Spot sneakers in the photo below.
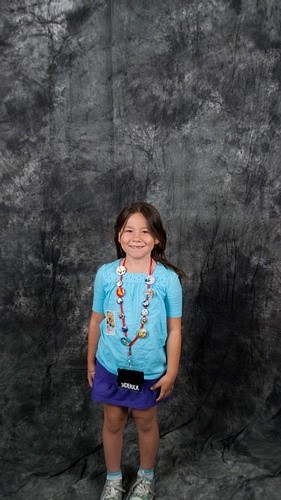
[100,475,125,500]
[127,478,155,500]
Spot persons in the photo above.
[88,201,192,500]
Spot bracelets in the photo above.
[87,371,96,373]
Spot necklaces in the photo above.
[115,256,155,355]
